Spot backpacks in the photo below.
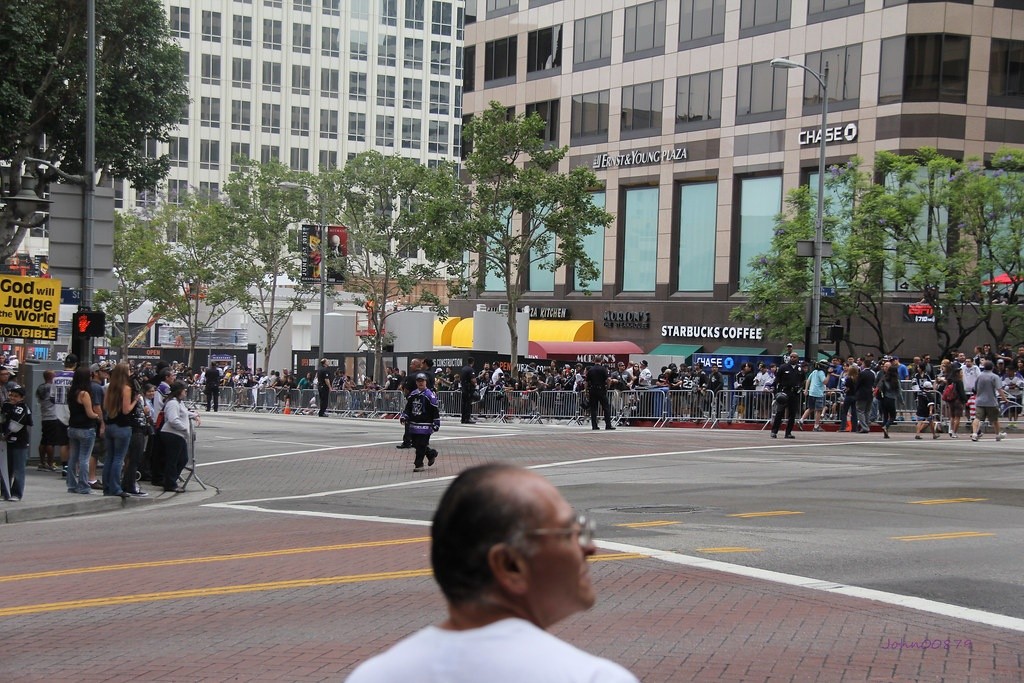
[942,380,957,402]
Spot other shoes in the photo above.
[396,443,411,449]
[948,430,958,438]
[414,466,425,472]
[971,433,979,441]
[460,419,476,424]
[969,432,983,437]
[915,435,923,439]
[428,452,438,466]
[317,411,328,417]
[60,471,186,498]
[0,495,21,502]
[769,422,889,438]
[933,435,940,439]
[592,426,616,430]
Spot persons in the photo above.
[987,286,1019,304]
[348,461,642,683]
[0,342,1024,502]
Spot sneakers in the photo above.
[996,432,1008,442]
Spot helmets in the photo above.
[574,363,583,369]
[776,393,789,406]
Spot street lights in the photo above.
[277,180,325,364]
[770,56,830,365]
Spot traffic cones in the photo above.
[283,399,290,415]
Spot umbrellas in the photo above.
[983,273,1024,291]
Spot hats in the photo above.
[866,353,899,361]
[435,368,442,374]
[65,353,111,373]
[640,360,648,366]
[787,342,793,347]
[416,373,428,381]
[923,381,933,389]
[7,381,25,396]
[997,359,1005,364]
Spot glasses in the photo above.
[621,365,625,366]
[528,516,595,549]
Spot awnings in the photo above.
[433,317,594,348]
[781,349,835,361]
[712,347,768,355]
[647,344,703,366]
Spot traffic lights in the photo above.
[827,324,844,341]
[72,310,105,338]
